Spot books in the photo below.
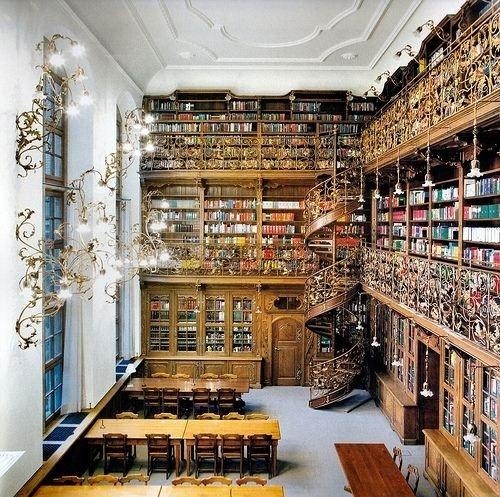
[149,197,315,270]
[147,100,375,135]
[149,299,251,351]
[444,346,500,484]
[377,173,500,268]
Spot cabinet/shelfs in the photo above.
[360,0,500,497]
[137,89,379,388]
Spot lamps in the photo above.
[374,70,397,86]
[420,338,435,398]
[393,146,405,195]
[422,126,436,188]
[358,165,365,203]
[391,318,402,367]
[373,156,383,199]
[289,91,296,101]
[467,102,484,177]
[463,368,483,448]
[412,19,452,44]
[393,44,420,64]
[345,91,354,101]
[14,107,183,350]
[224,93,232,101]
[363,85,388,102]
[14,33,96,178]
[370,305,381,347]
[356,293,364,329]
[169,94,177,101]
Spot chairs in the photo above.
[31,372,284,497]
[393,446,403,472]
[406,464,421,495]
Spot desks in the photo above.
[334,443,416,497]
